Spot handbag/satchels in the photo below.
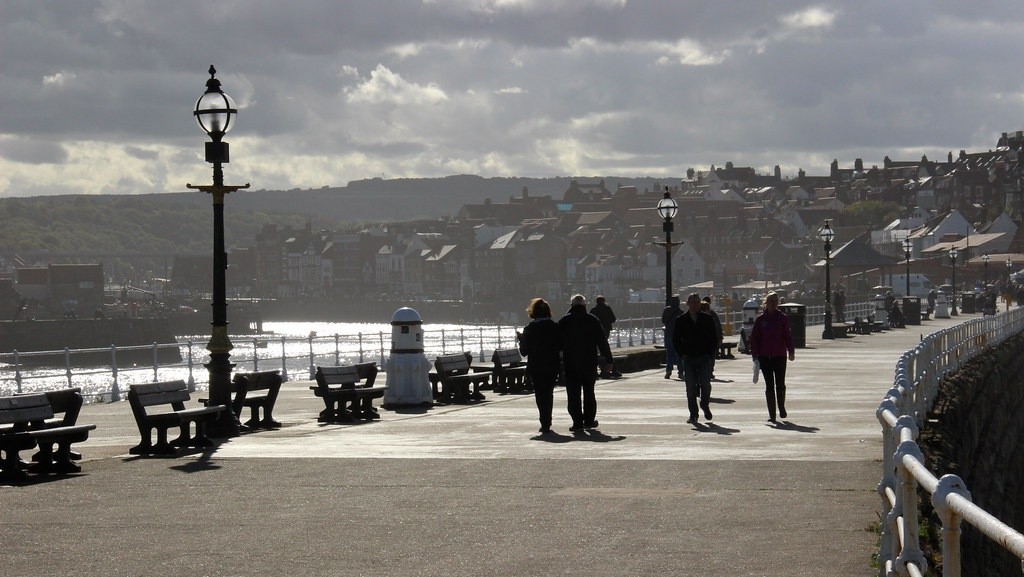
[753,359,760,384]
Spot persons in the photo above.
[662,293,722,423]
[589,297,616,339]
[750,292,795,422]
[884,279,1024,328]
[832,290,847,323]
[517,297,558,432]
[556,294,613,431]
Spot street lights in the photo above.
[652,185,683,308]
[902,236,914,297]
[186,62,251,438]
[949,245,958,316]
[819,219,836,340]
[1006,256,1013,306]
[983,250,990,292]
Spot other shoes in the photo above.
[687,414,698,423]
[539,422,550,433]
[612,370,622,377]
[678,371,684,379]
[711,373,715,379]
[569,425,583,431]
[584,419,598,429]
[665,371,671,378]
[700,401,712,420]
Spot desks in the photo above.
[470,348,510,391]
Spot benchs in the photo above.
[128,380,227,458]
[314,366,390,424]
[428,350,472,400]
[844,314,884,335]
[716,342,738,359]
[0,392,97,486]
[0,388,83,470]
[921,307,933,320]
[494,348,535,394]
[310,361,378,417]
[196,370,283,432]
[433,354,493,404]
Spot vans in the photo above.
[879,274,938,298]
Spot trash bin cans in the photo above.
[902,296,921,325]
[778,304,806,348]
[962,293,975,314]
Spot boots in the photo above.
[765,390,776,423]
[776,386,787,418]
